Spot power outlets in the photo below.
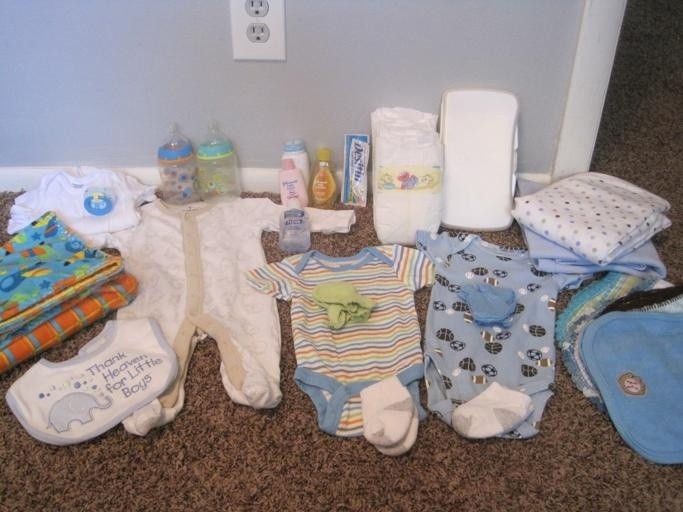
[229,0,286,60]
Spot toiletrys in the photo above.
[309,149,338,210]
[280,159,308,208]
[279,198,310,252]
